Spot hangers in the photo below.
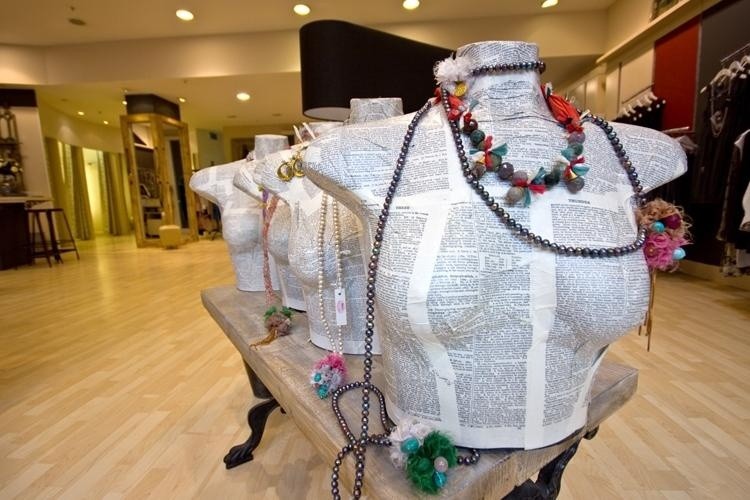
[659,127,699,155]
[612,83,667,121]
[700,43,750,94]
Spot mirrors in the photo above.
[119,113,199,248]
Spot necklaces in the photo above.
[331,61,647,499]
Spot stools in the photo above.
[25,208,79,268]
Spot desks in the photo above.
[201,286,639,500]
